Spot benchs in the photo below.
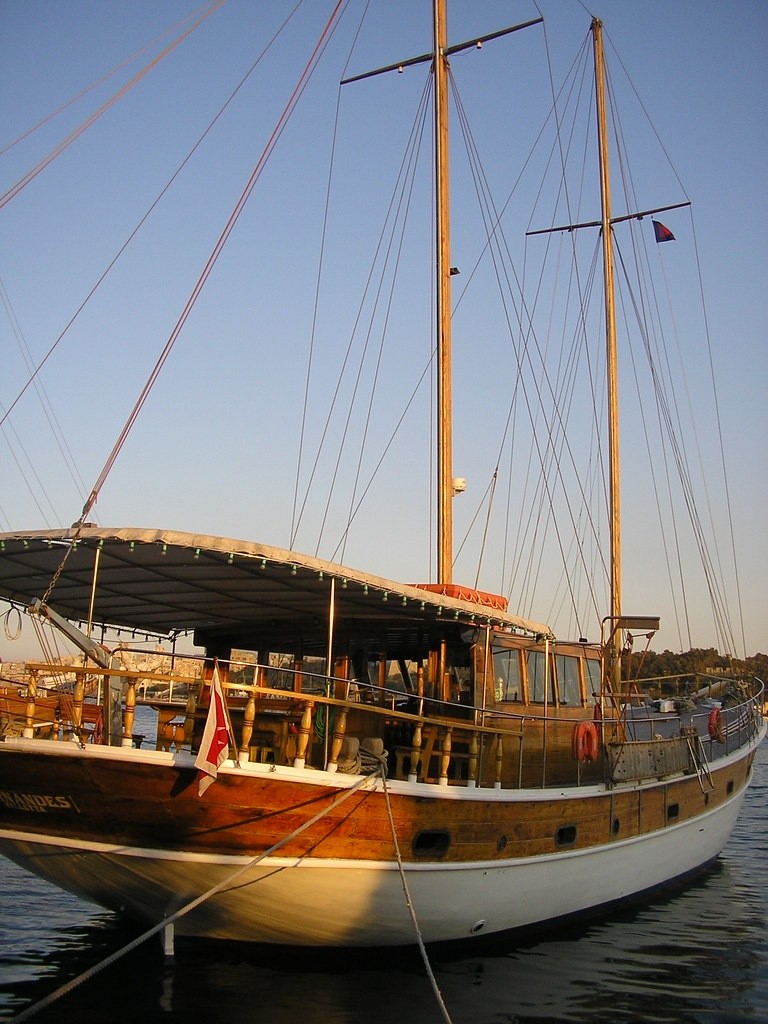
[60,701,145,749]
[390,714,496,788]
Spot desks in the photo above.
[134,701,304,765]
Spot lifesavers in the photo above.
[574,721,600,761]
[707,706,723,740]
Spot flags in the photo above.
[652,220,676,243]
[194,668,230,797]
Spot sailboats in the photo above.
[2,1,768,947]
